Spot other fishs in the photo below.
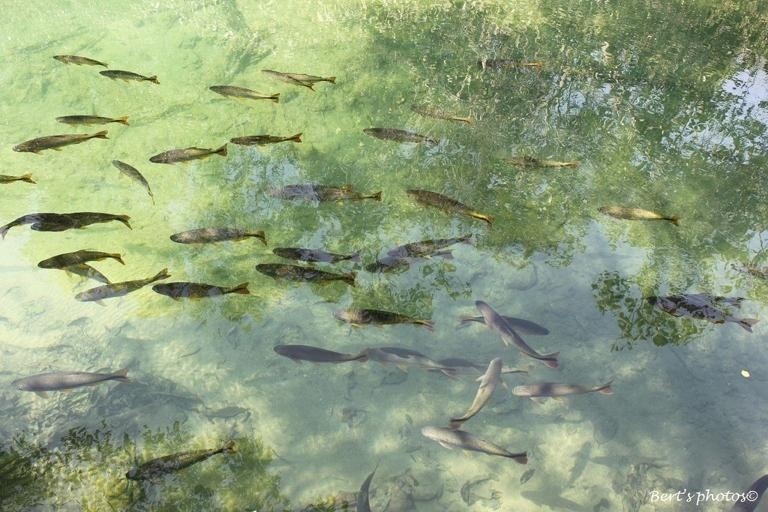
[0,52,767,512]
